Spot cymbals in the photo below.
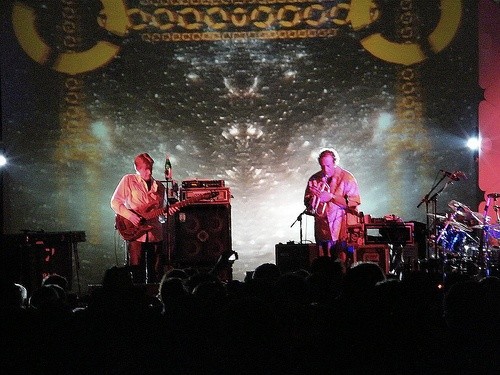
[473,211,495,223]
[470,224,488,230]
[447,199,469,217]
[427,212,446,220]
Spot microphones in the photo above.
[441,170,460,182]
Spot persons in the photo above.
[0,249,500,375]
[110,152,180,266]
[305,151,360,265]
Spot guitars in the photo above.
[115,191,219,241]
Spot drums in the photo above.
[463,244,500,267]
[437,222,471,256]
[483,224,500,243]
[438,254,475,290]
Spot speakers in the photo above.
[168,203,231,266]
[105,263,145,287]
[276,244,320,273]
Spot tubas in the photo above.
[303,173,331,218]
